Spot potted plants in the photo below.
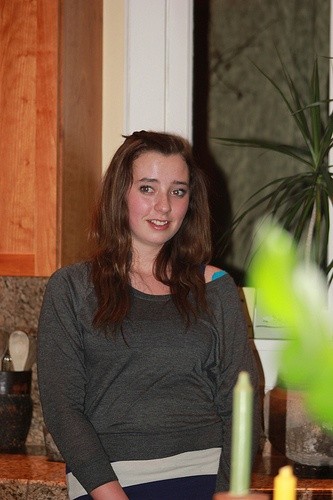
[211,33,332,482]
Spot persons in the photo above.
[37,130,259,500]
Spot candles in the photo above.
[272,464,297,499]
[225,371,253,496]
